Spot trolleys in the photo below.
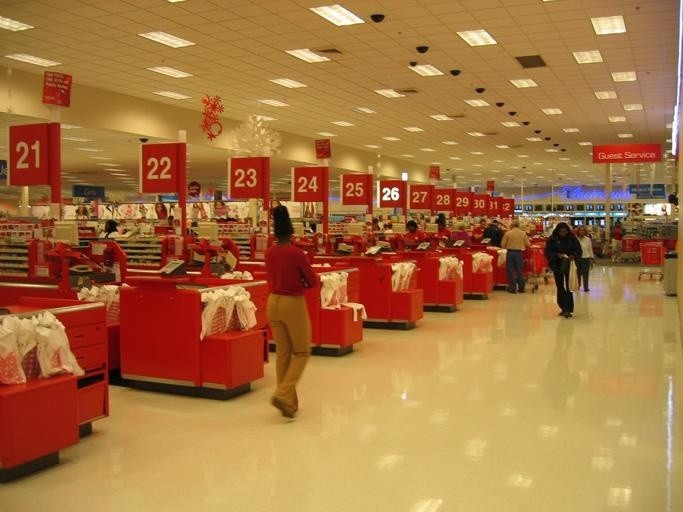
[620,234,642,263]
[637,241,665,282]
[519,239,552,292]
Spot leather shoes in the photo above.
[559,311,570,317]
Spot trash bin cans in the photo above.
[664,250,678,296]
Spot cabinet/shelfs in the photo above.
[1,214,469,274]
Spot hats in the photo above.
[273,205,294,236]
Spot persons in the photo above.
[544,223,582,317]
[400,221,428,243]
[612,221,622,263]
[501,220,531,293]
[472,217,502,247]
[99,221,120,239]
[574,225,594,291]
[429,218,452,239]
[264,216,320,418]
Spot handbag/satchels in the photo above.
[560,257,571,273]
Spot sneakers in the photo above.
[272,397,295,418]
[509,290,525,293]
